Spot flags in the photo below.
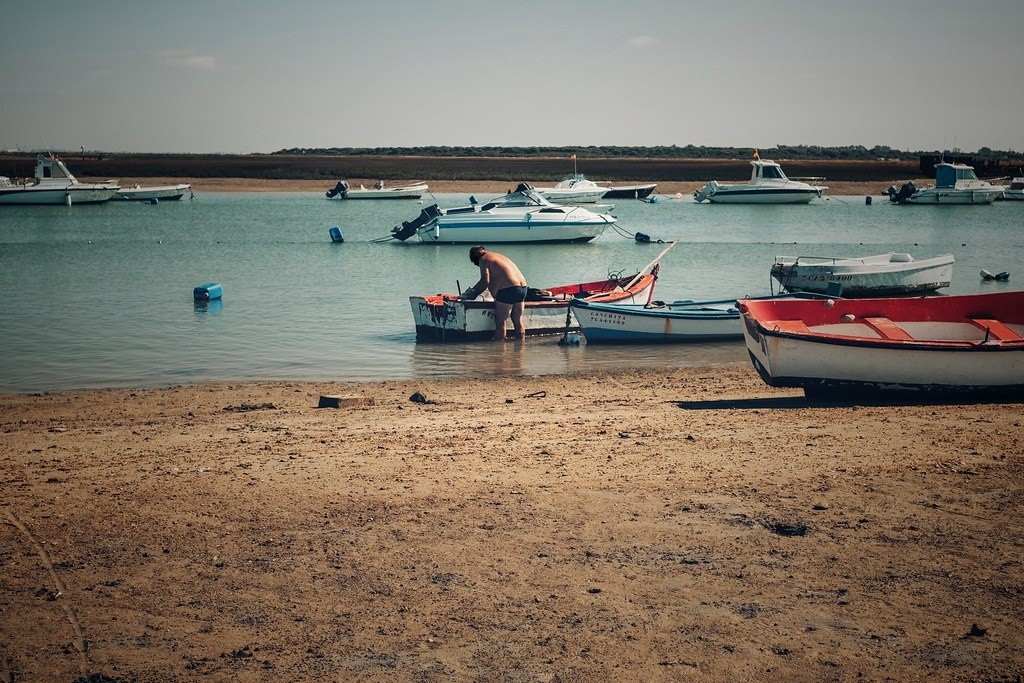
[754,153,758,157]
[571,154,575,159]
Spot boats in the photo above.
[110,183,190,201]
[881,162,1007,206]
[569,297,746,342]
[0,156,121,202]
[393,187,617,243]
[604,183,658,198]
[507,154,614,203]
[327,179,429,199]
[692,147,829,204]
[735,291,1024,388]
[408,263,661,337]
[769,249,955,295]
[994,177,1024,199]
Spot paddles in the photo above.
[613,239,679,294]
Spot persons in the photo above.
[457,245,527,340]
